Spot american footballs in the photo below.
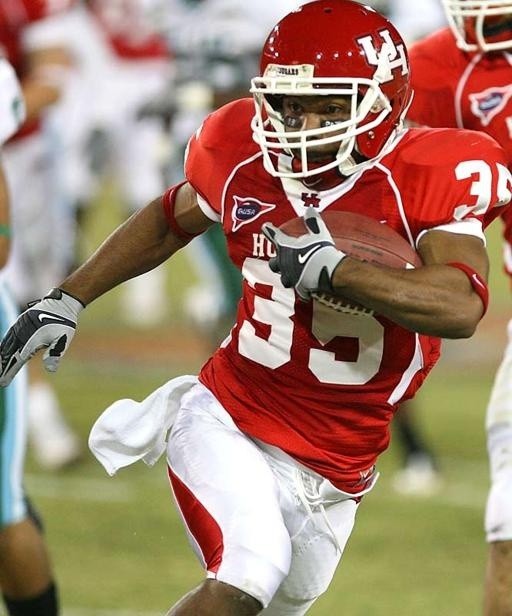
[277,211,424,319]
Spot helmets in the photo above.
[443,2,511,53]
[250,0,412,180]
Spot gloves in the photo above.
[0,289,85,387]
[262,207,345,303]
